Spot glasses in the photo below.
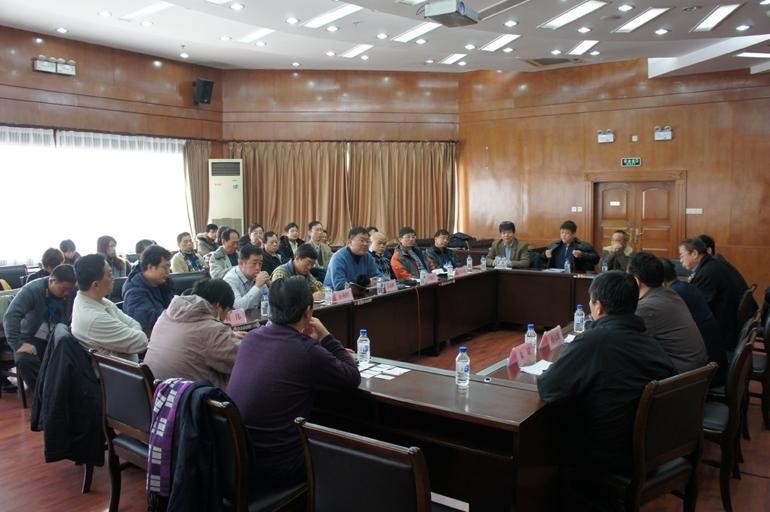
[352,238,373,245]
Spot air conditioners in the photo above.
[207,158,244,243]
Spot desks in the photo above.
[328,245,345,253]
[574,272,607,316]
[317,279,441,360]
[472,265,573,327]
[421,264,498,357]
[231,299,352,348]
[476,313,592,393]
[417,247,491,266]
[348,354,549,512]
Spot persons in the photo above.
[240,225,252,246]
[425,229,461,273]
[240,224,264,250]
[260,231,290,275]
[486,221,531,267]
[26,248,65,283]
[223,244,270,312]
[0,278,11,385]
[598,230,635,272]
[368,232,390,282]
[390,226,474,347]
[270,243,324,300]
[3,264,77,395]
[132,239,172,273]
[279,223,305,264]
[203,225,230,257]
[121,244,175,338]
[697,235,759,321]
[626,252,708,376]
[196,224,219,256]
[678,237,738,349]
[367,226,391,259]
[226,276,360,484]
[209,228,240,280]
[305,221,333,281]
[540,220,600,273]
[537,270,681,484]
[656,256,729,389]
[321,230,327,242]
[60,239,81,265]
[171,232,210,274]
[323,227,386,291]
[71,254,147,364]
[138,278,249,448]
[97,236,126,277]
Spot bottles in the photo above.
[265,315,273,326]
[564,258,570,273]
[480,255,486,269]
[420,269,427,285]
[325,284,333,305]
[448,261,453,279]
[377,274,384,294]
[601,260,608,272]
[525,324,537,359]
[466,255,472,271]
[456,347,470,386]
[261,292,269,316]
[357,329,370,363]
[574,304,585,334]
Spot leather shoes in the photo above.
[461,333,472,341]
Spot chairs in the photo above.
[606,361,718,512]
[309,265,329,285]
[294,417,432,512]
[0,249,210,310]
[88,348,155,512]
[153,379,308,512]
[52,323,109,494]
[0,295,34,409]
[683,328,757,512]
[707,284,770,464]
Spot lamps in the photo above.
[597,129,614,143]
[654,126,672,140]
[33,55,76,75]
